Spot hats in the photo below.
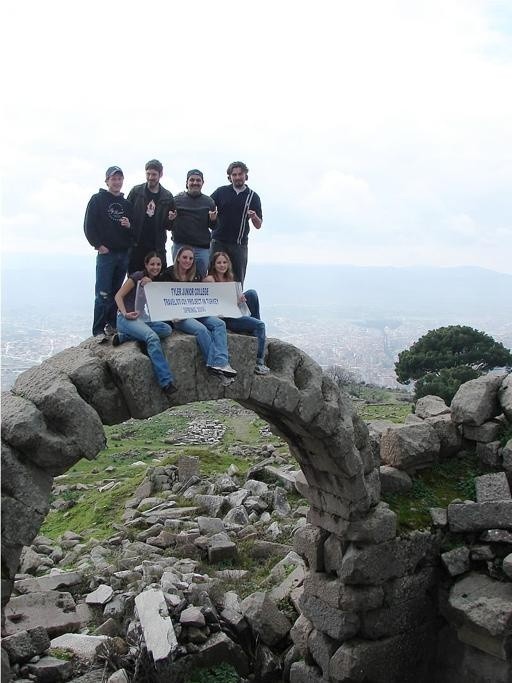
[106,166,123,178]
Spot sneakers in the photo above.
[94,323,120,346]
[206,359,237,387]
[165,383,177,393]
[255,365,269,375]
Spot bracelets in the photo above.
[125,313,127,318]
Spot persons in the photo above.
[110,250,180,395]
[207,161,263,294]
[171,167,222,279]
[158,244,238,386]
[199,252,273,376]
[127,158,178,280]
[84,164,135,346]
